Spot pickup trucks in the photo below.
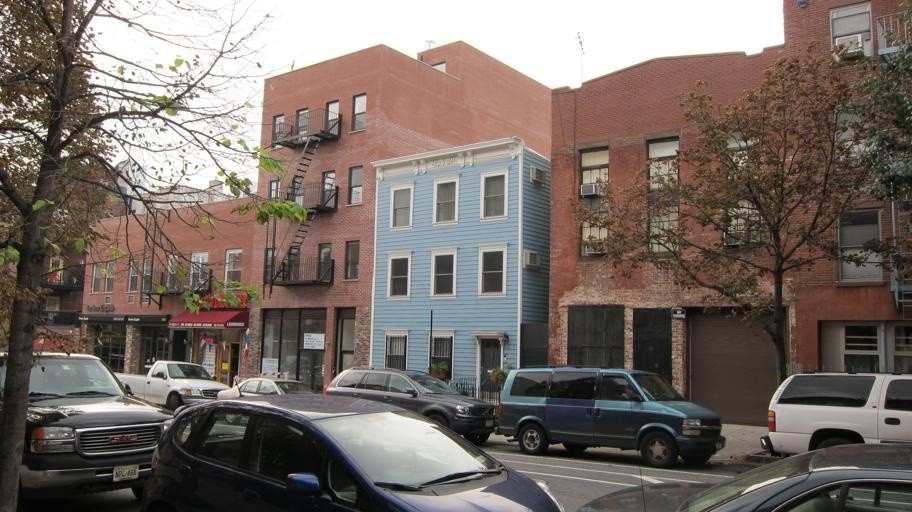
[109,359,231,413]
[1,351,174,512]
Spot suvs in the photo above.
[758,370,912,456]
[323,365,494,448]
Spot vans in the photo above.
[493,365,729,470]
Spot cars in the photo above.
[571,438,911,512]
[146,392,564,510]
[216,378,317,424]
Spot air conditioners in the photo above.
[579,183,600,198]
[835,34,864,62]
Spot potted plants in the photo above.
[431,360,449,379]
